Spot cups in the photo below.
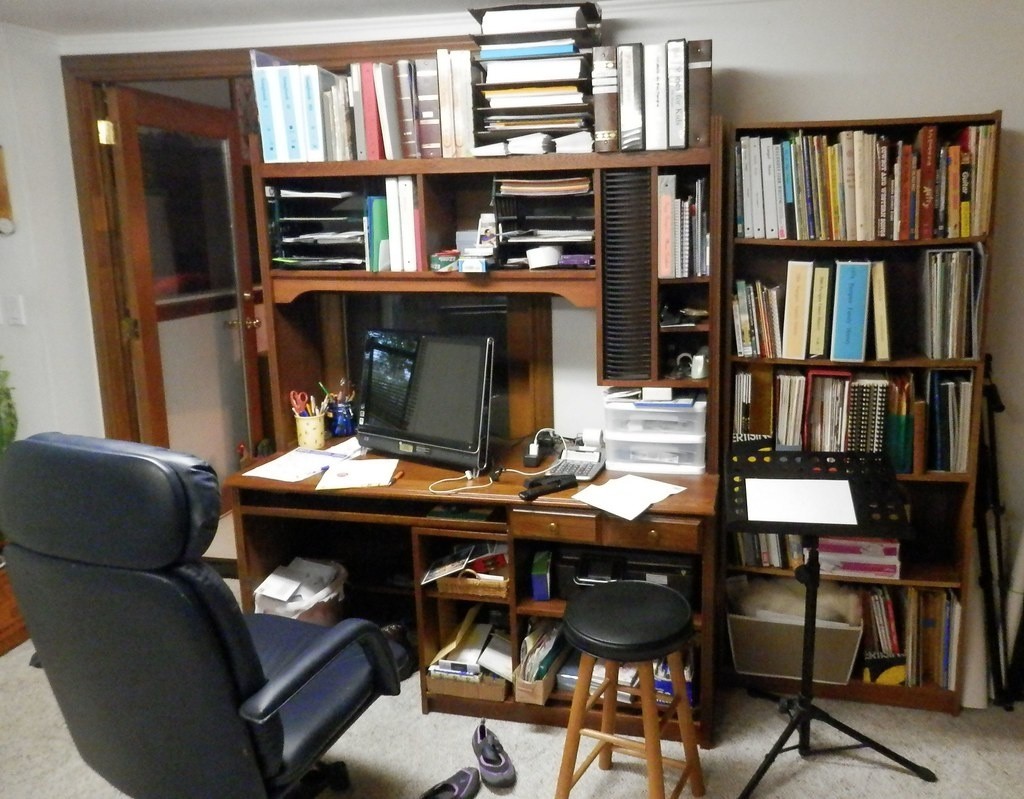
[327,401,355,435]
[294,412,325,449]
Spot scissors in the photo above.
[290,391,308,413]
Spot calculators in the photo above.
[544,445,607,482]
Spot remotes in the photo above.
[524,474,576,489]
[519,480,579,501]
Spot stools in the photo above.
[555,581,707,799]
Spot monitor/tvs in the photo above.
[356,327,496,471]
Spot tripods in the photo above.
[721,451,938,799]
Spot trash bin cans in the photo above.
[255,557,350,630]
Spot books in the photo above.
[256,555,338,609]
[734,529,902,580]
[424,599,513,684]
[731,123,998,240]
[494,174,594,269]
[589,649,696,712]
[363,175,427,275]
[727,246,978,474]
[252,3,712,164]
[857,585,963,689]
[657,172,714,279]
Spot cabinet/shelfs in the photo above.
[717,109,1005,717]
[247,113,726,474]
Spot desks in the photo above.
[223,437,722,750]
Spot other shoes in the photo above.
[472,717,516,787]
[419,768,479,799]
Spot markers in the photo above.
[336,377,345,403]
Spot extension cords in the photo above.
[524,445,547,467]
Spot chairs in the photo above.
[0,430,418,799]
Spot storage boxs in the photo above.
[511,642,575,706]
[438,656,485,673]
[602,389,707,435]
[726,607,865,687]
[601,431,707,474]
[651,646,701,707]
[530,550,552,602]
[424,673,510,702]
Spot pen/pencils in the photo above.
[346,391,356,401]
[386,471,403,487]
[319,382,336,404]
[292,395,330,417]
